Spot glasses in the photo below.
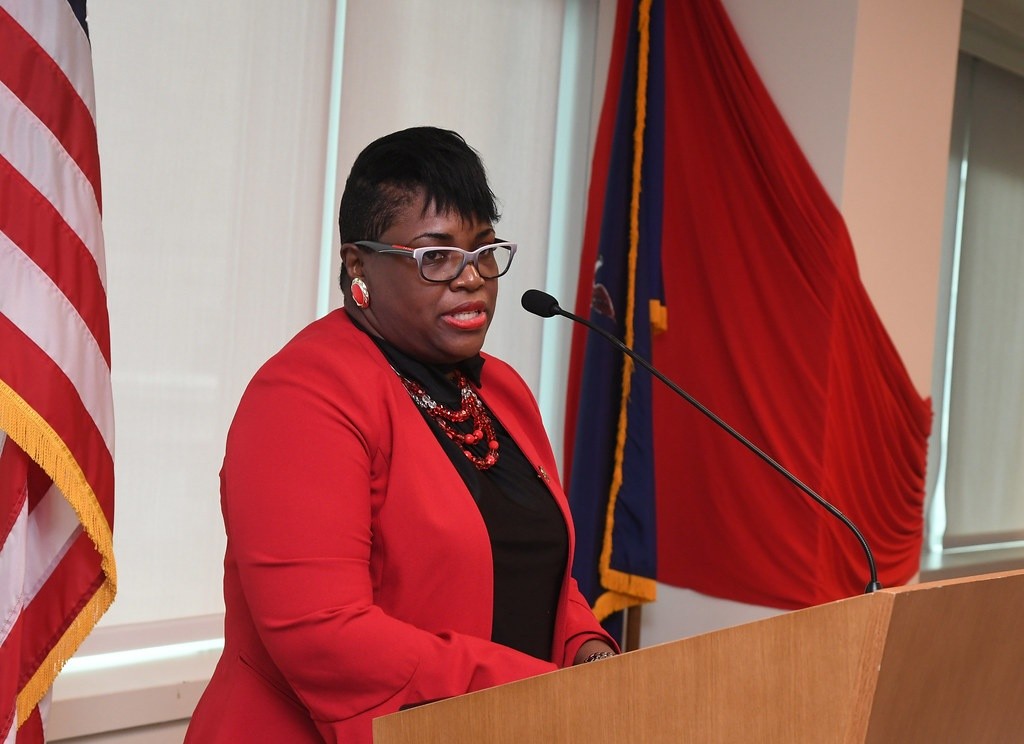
[353,237,518,285]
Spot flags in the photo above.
[0,0,116,744]
[564,0,665,624]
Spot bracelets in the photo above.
[584,653,615,663]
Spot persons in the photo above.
[181,126,619,744]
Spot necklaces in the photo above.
[389,356,499,472]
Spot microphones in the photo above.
[521,289,883,595]
